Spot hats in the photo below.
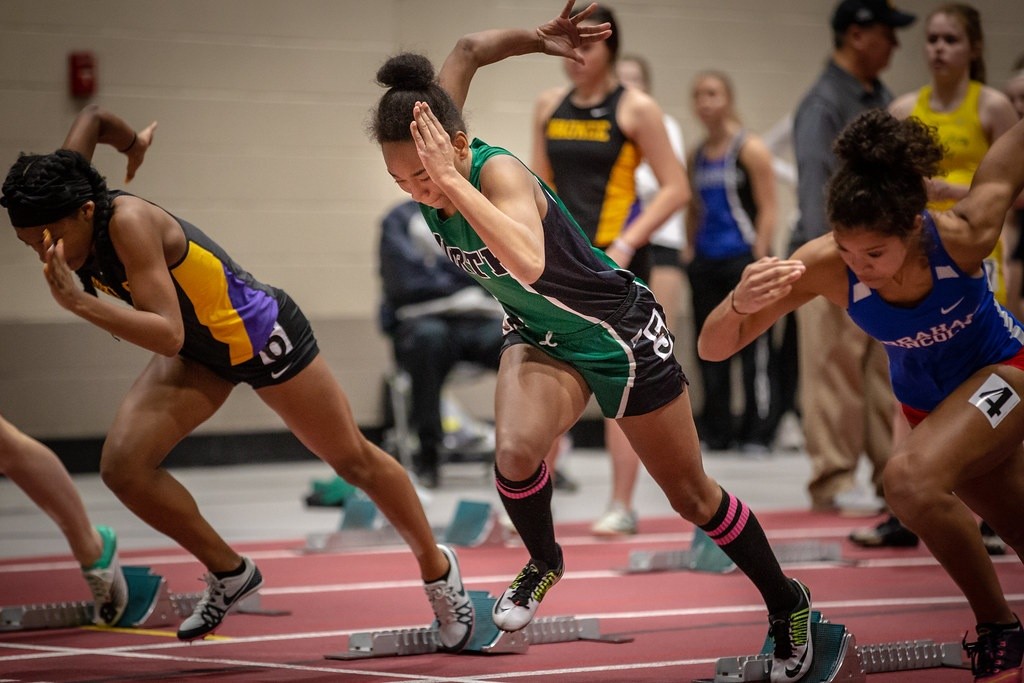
[828,0,917,30]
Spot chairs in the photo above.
[379,299,493,475]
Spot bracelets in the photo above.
[615,239,635,255]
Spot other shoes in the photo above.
[704,414,738,451]
[592,507,640,535]
[738,412,774,452]
[411,452,439,488]
[551,461,577,492]
[776,411,805,449]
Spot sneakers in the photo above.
[420,544,477,650]
[979,519,1008,554]
[179,557,266,642]
[79,524,130,626]
[851,515,918,548]
[767,578,812,683]
[960,612,1024,683]
[493,542,567,632]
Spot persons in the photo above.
[0,416,128,626]
[620,56,803,451]
[378,200,577,492]
[698,111,1024,683]
[794,0,1023,553]
[375,0,815,683]
[527,7,693,537]
[0,104,475,652]
